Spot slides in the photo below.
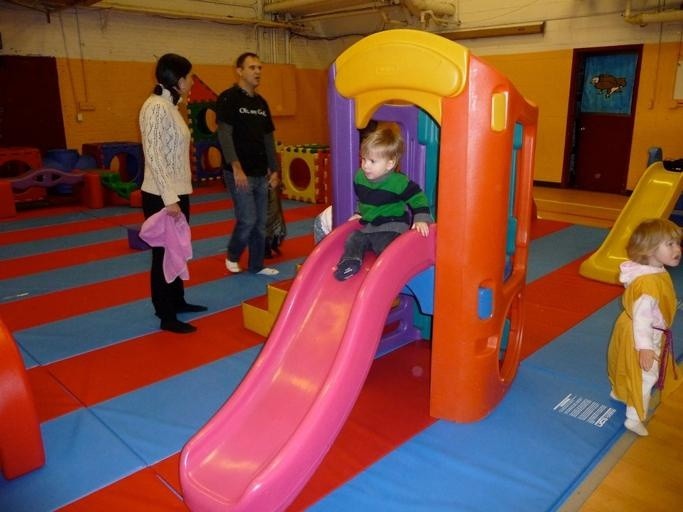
[581,160,683,287]
[179,218,436,511]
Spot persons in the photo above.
[334,128,432,281]
[139,53,208,334]
[607,218,683,436]
[215,52,281,277]
[313,205,333,246]
